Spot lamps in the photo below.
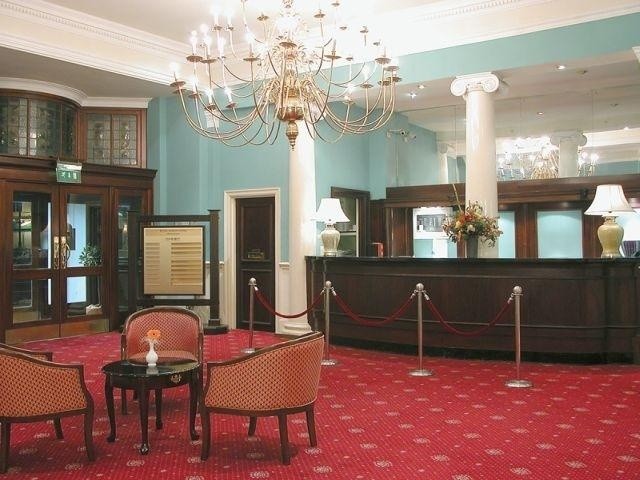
[584,184,636,258]
[168,1,403,151]
[496,136,599,181]
[311,198,350,257]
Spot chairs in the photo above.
[121,306,325,465]
[0,340,98,473]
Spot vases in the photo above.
[466,238,477,258]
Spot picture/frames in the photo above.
[412,207,453,240]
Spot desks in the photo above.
[101,357,203,455]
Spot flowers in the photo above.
[440,200,504,249]
